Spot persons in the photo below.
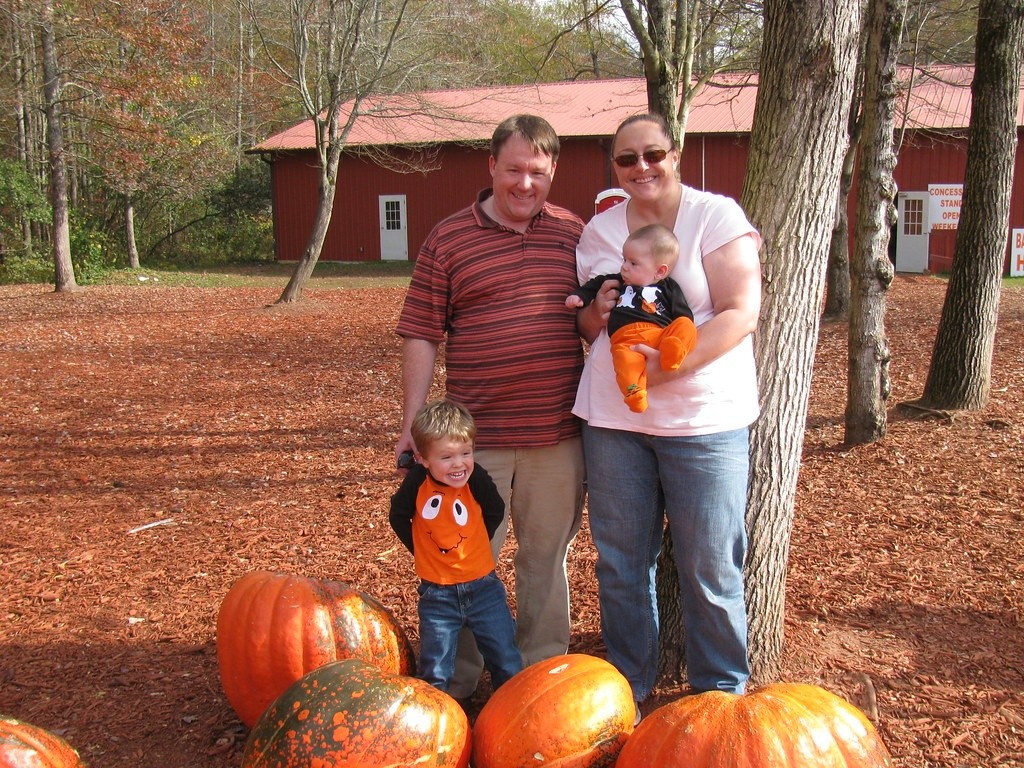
[564,222,698,415]
[571,112,764,728]
[387,398,525,694]
[392,112,586,701]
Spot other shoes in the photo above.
[453,696,469,713]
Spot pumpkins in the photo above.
[216,569,417,730]
[240,659,473,768]
[0,714,92,768]
[617,683,896,768]
[466,654,636,768]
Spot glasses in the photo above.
[613,147,673,167]
